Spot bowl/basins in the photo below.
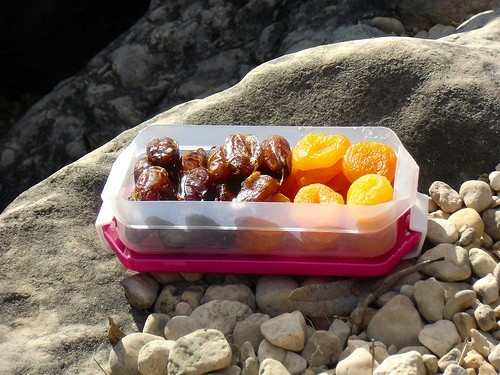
[94,125,430,277]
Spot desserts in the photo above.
[238,133,397,254]
